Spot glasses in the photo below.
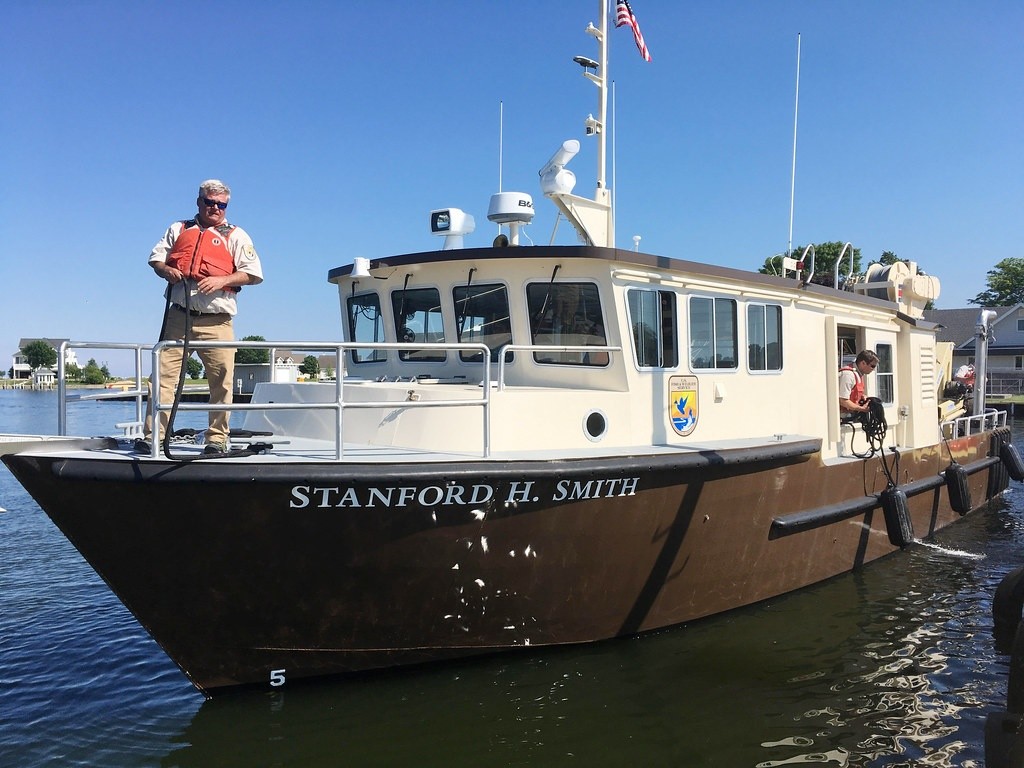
[867,363,876,369]
[199,197,228,209]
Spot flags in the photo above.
[615,0,652,63]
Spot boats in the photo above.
[2,0,1014,700]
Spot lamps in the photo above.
[350,257,371,278]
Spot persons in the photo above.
[838,349,880,419]
[132,178,265,457]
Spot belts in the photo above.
[172,303,218,317]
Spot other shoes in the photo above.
[133,441,151,453]
[204,438,227,455]
